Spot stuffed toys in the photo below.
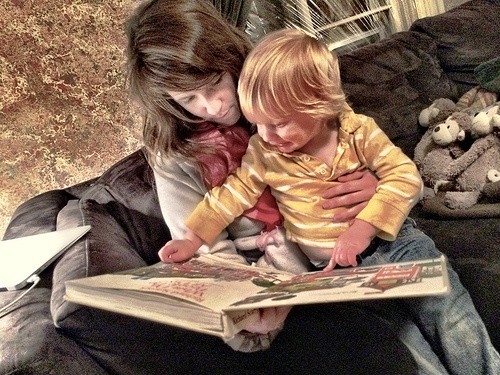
[414,99,500,210]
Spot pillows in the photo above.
[49,200,184,370]
[335,27,458,155]
[409,0,500,106]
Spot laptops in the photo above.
[0,225,93,290]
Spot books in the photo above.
[62,252,451,340]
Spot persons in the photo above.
[157,27,500,375]
[123,1,314,353]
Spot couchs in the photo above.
[0,0,500,374]
